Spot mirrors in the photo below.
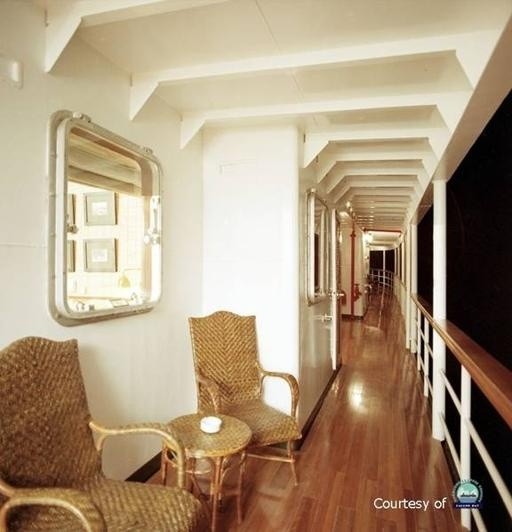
[308,192,329,304]
[56,112,163,324]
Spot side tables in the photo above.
[160,413,252,532]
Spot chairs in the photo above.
[0,335,206,532]
[188,311,303,507]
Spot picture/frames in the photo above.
[66,190,117,274]
